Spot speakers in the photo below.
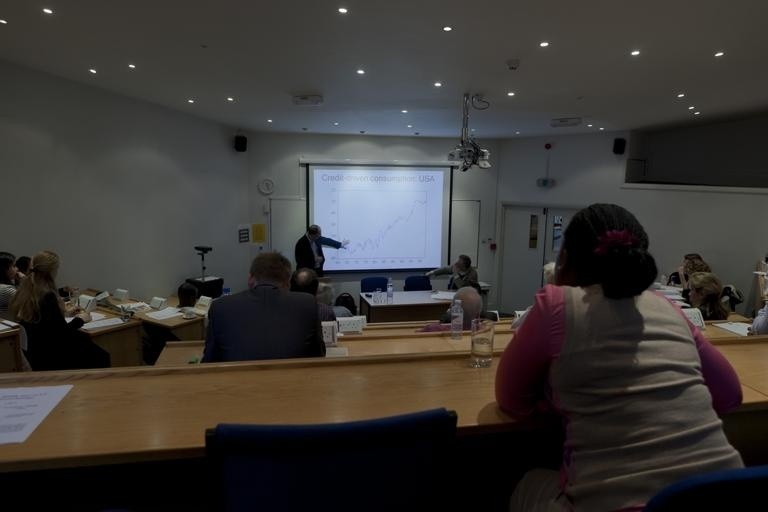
[235,135,247,152]
[612,138,626,155]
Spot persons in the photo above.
[544,262,556,286]
[177,282,198,309]
[679,253,768,336]
[200,225,358,363]
[495,202,745,512]
[0,250,111,371]
[425,255,478,290]
[415,286,483,332]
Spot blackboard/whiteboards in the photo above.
[268,197,481,271]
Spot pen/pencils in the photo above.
[78,298,79,312]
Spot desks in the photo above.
[0,274,767,478]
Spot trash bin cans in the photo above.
[477,281,491,313]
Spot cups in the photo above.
[184,309,194,316]
[372,287,385,302]
[68,287,81,307]
[652,282,661,289]
[467,317,495,369]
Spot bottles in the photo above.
[387,278,393,298]
[450,298,463,339]
[661,273,667,287]
[223,287,231,294]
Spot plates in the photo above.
[183,315,198,320]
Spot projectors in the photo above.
[447,147,492,172]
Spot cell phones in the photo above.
[365,293,372,297]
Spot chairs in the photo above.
[202,408,459,512]
[639,465,768,510]
